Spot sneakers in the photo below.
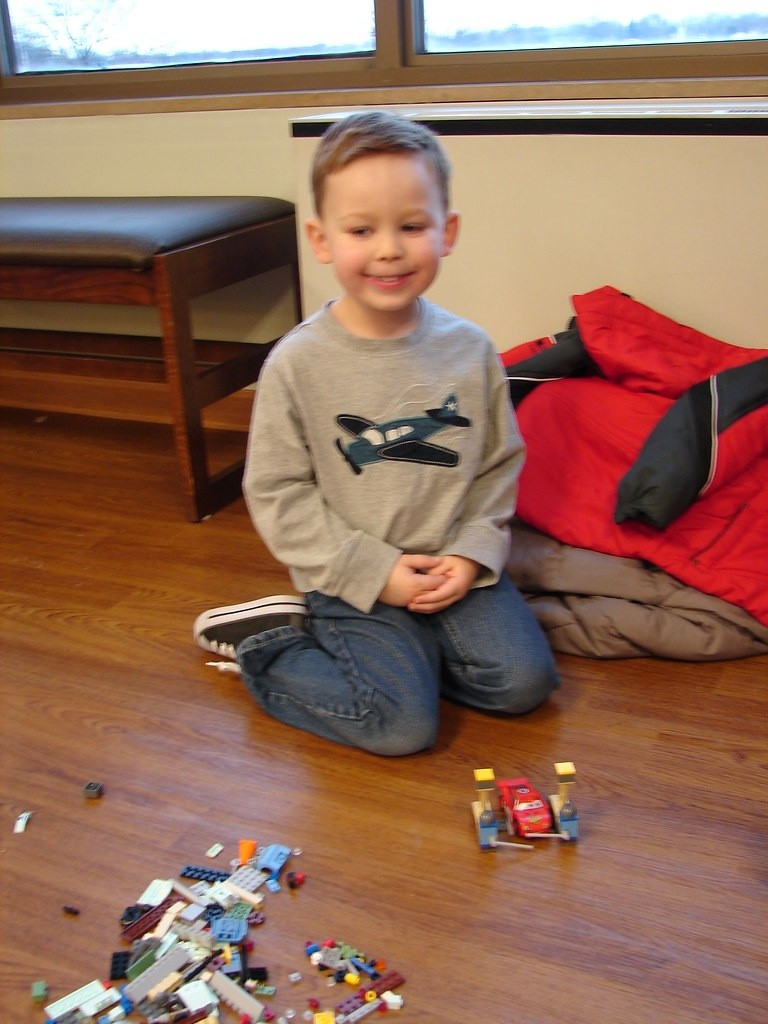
[192,595,308,674]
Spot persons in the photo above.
[194,112,562,756]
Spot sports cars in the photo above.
[496,777,552,836]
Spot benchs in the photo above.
[0,197,305,522]
